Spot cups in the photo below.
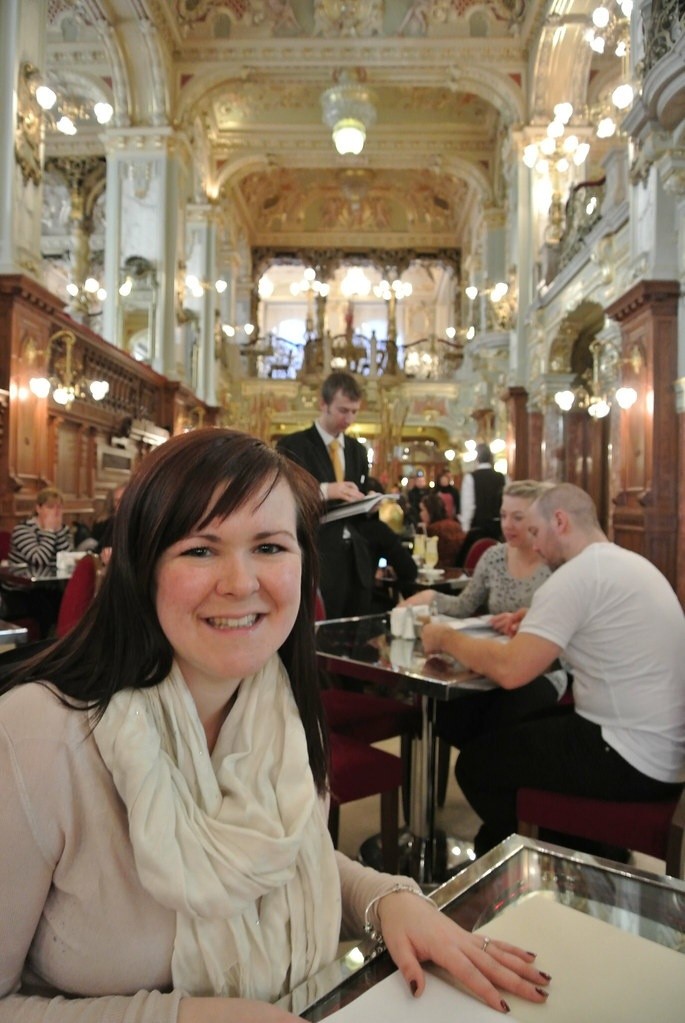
[412,555,423,568]
[425,537,438,568]
[412,534,427,566]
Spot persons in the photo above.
[1,491,74,640]
[408,475,465,566]
[396,480,554,642]
[0,429,553,1023]
[421,482,684,857]
[92,484,130,566]
[276,371,405,617]
[452,443,506,567]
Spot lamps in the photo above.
[68,256,152,316]
[21,329,110,405]
[26,59,116,135]
[317,84,379,156]
[176,274,257,338]
[522,0,635,170]
[553,340,650,421]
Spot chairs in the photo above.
[455,528,502,572]
[313,590,413,876]
[55,554,96,638]
[0,532,40,642]
[515,785,685,878]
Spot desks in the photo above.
[271,832,685,1023]
[313,612,562,888]
[375,565,470,593]
[0,619,28,653]
[0,565,65,592]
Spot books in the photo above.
[320,488,400,527]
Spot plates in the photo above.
[418,569,445,575]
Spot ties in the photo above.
[328,439,344,483]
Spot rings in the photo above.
[482,938,490,950]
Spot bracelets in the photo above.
[363,886,438,945]
